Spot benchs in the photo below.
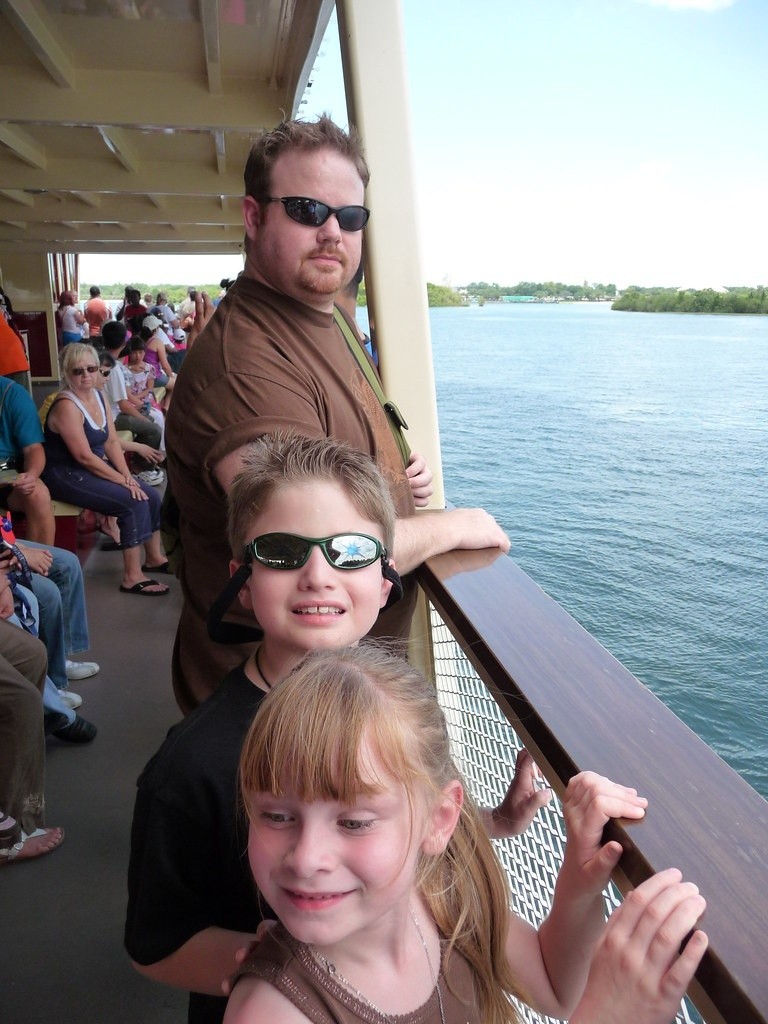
[10,386,167,572]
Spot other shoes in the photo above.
[57,689,83,710]
[164,459,168,468]
[161,408,167,417]
[64,657,100,680]
[51,713,98,745]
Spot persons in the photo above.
[267,549,307,566]
[162,114,509,716]
[0,311,99,861]
[38,262,372,594]
[327,545,341,561]
[291,199,317,220]
[123,428,554,1024]
[222,645,707,1024]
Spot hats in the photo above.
[102,321,133,347]
[172,328,186,341]
[142,315,163,332]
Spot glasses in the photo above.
[259,195,371,233]
[241,531,390,570]
[99,369,111,377]
[70,366,99,375]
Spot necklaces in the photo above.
[309,904,445,1024]
[84,401,98,415]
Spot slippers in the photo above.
[141,559,176,575]
[101,541,124,552]
[119,578,170,596]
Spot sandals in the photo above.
[0,825,66,865]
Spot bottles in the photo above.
[79,324,84,338]
[139,390,153,417]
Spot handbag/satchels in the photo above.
[159,478,187,579]
[0,509,17,546]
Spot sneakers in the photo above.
[128,468,165,486]
